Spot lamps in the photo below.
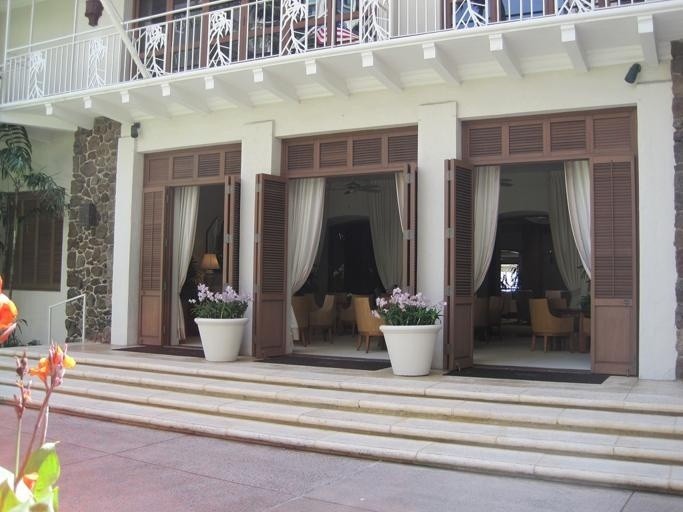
[200,253,221,275]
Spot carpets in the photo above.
[256,354,391,371]
[445,365,610,384]
[112,345,204,359]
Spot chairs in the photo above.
[477,290,575,352]
[292,292,386,354]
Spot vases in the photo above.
[193,318,247,362]
[379,326,439,376]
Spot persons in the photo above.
[500,275,511,288]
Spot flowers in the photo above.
[372,286,449,325]
[190,283,252,318]
